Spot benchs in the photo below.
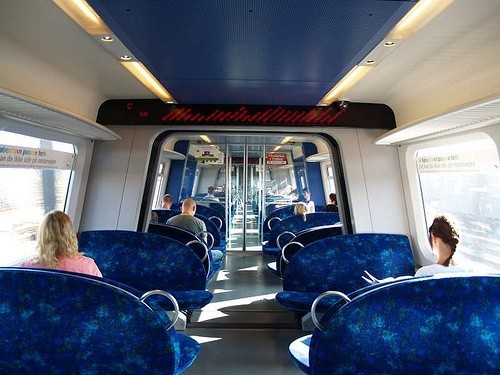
[252,190,500,375]
[0,192,239,375]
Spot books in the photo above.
[362,269,413,285]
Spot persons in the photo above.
[19,209,105,279]
[166,198,224,291]
[150,212,159,224]
[413,213,474,277]
[162,194,173,210]
[288,186,340,222]
[202,186,220,202]
